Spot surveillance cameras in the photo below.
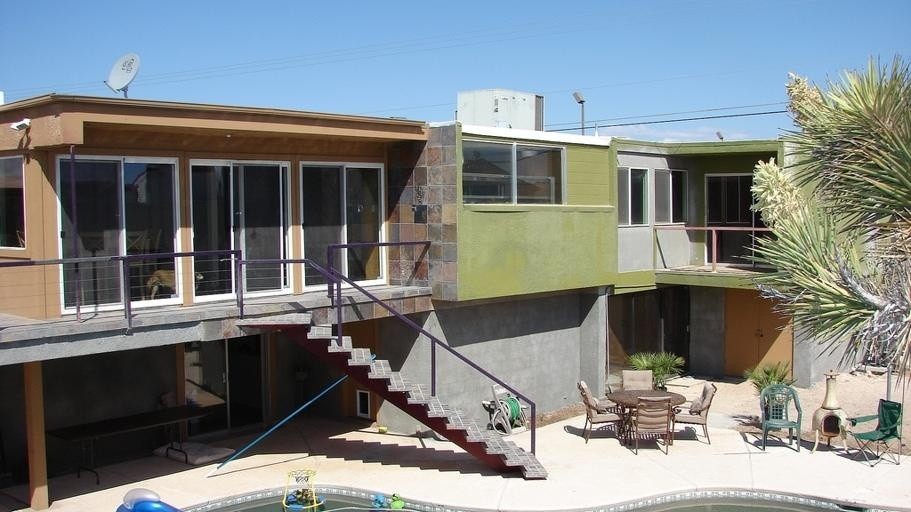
[572,92,586,106]
[715,132,724,141]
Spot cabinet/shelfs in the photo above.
[462,172,555,202]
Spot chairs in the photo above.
[845,399,902,467]
[760,384,801,452]
[78,229,161,301]
[576,370,716,455]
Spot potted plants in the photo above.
[626,351,685,392]
[747,361,798,420]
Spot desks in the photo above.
[48,403,211,486]
